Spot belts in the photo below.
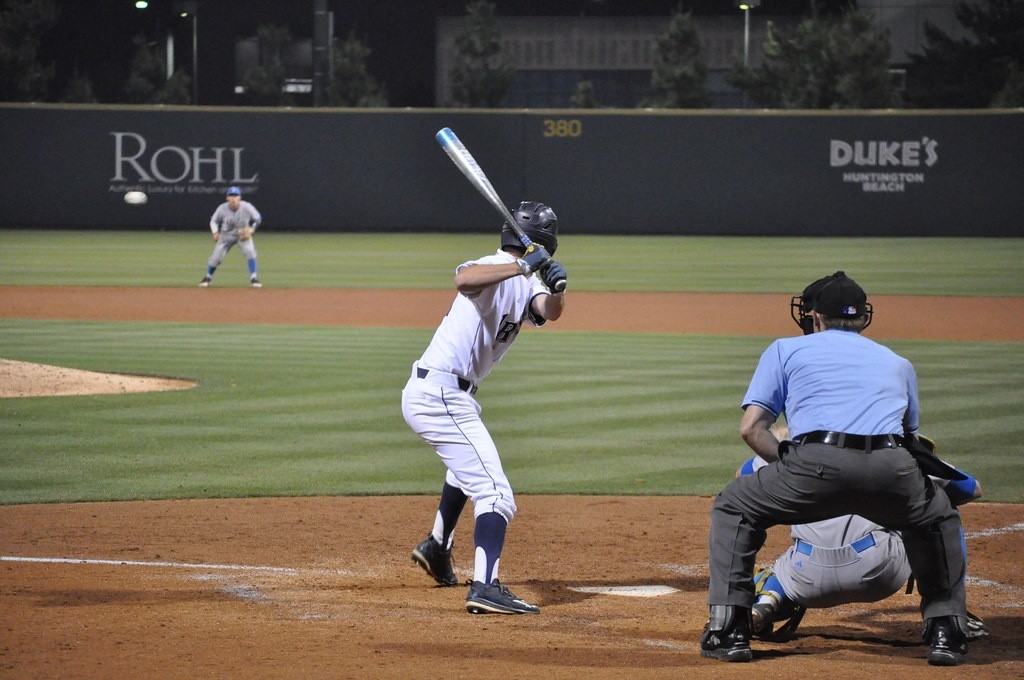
[792,431,907,449]
[796,533,875,556]
[417,367,477,394]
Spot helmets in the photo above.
[500,201,558,256]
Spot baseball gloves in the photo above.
[238,226,254,241]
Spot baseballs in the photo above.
[124,190,148,205]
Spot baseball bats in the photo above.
[434,125,569,294]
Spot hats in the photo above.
[803,276,867,318]
[227,187,241,195]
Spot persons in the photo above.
[750,437,987,640]
[401,201,568,615]
[699,272,972,665]
[199,187,262,287]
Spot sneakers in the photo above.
[466,579,540,615]
[701,608,753,661]
[412,533,458,586]
[751,603,775,636]
[928,616,968,665]
[967,619,989,638]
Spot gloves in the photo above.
[516,243,551,277]
[540,259,566,296]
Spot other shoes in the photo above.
[252,278,263,288]
[198,276,211,286]
